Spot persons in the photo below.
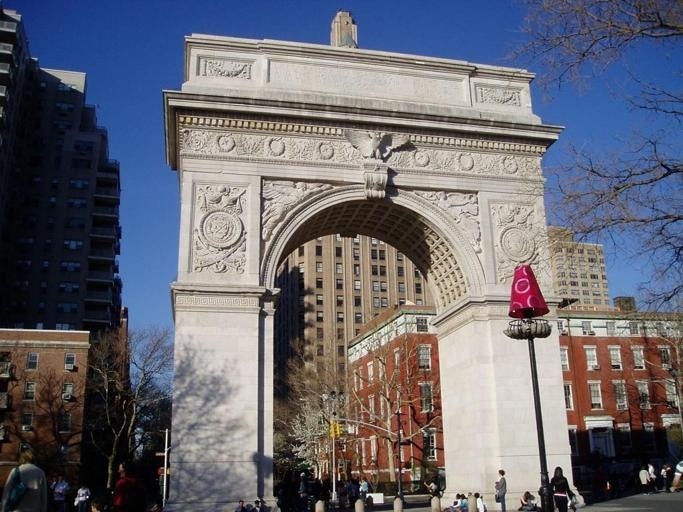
[443,492,488,512]
[495,470,506,512]
[518,491,537,512]
[423,469,442,498]
[639,461,683,496]
[235,500,248,512]
[273,467,369,512]
[550,467,576,512]
[1,447,137,512]
[250,500,264,512]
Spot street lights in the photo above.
[505,265,556,511]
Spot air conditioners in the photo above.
[588,331,595,335]
[62,392,71,399]
[65,364,74,370]
[561,329,568,335]
[592,365,600,370]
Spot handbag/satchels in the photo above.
[4,482,27,512]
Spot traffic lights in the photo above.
[330,421,339,439]
